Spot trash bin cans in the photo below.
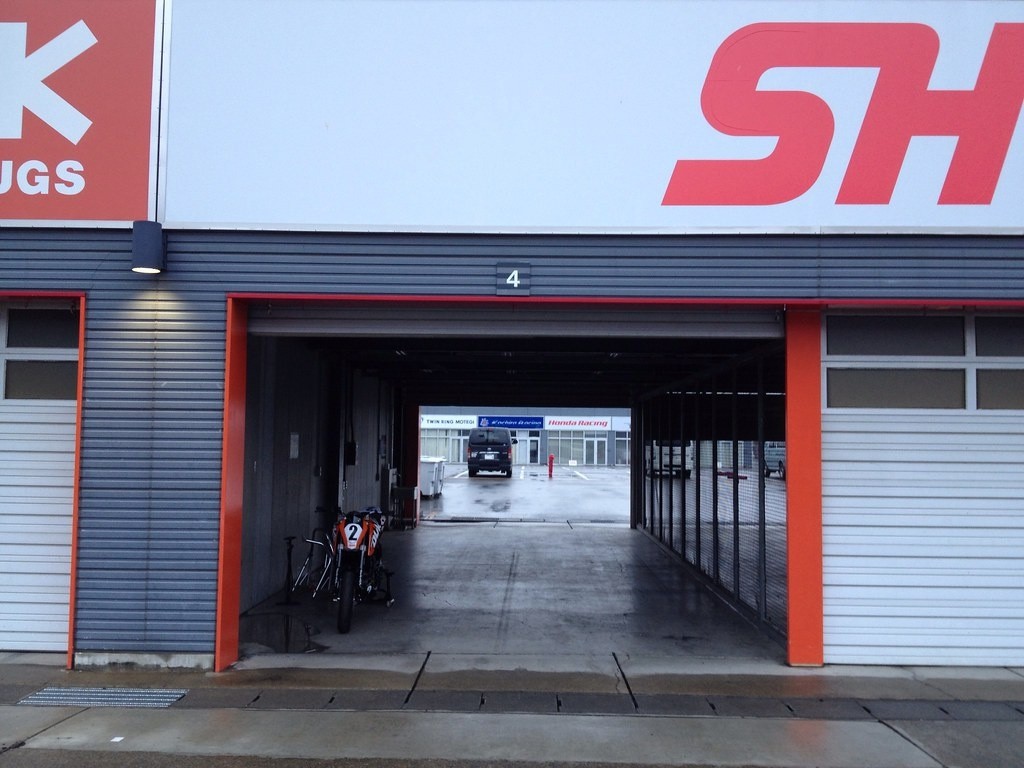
[419,456,449,499]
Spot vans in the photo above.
[646,436,693,479]
[762,439,788,479]
[466,427,513,479]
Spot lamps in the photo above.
[131,221,168,275]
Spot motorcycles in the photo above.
[316,503,390,634]
